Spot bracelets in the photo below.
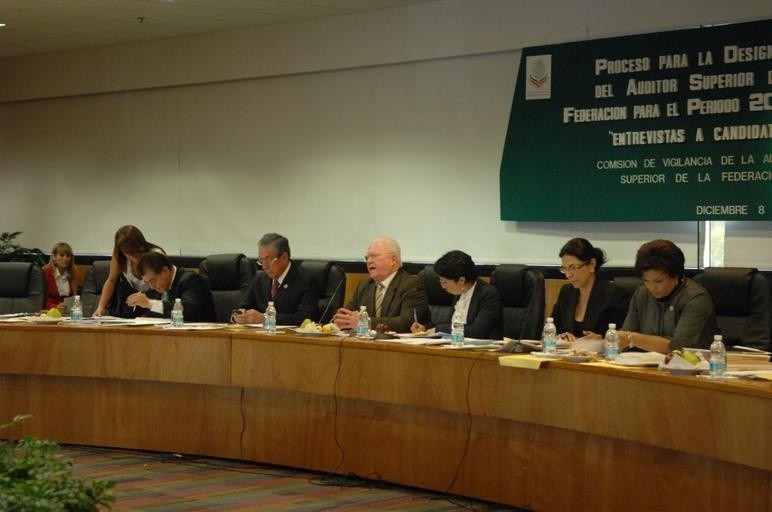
[627,332,635,349]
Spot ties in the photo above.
[271,279,281,299]
[374,284,383,317]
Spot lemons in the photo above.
[315,326,322,333]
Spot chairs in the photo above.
[609,277,644,300]
[490,263,545,341]
[414,267,461,326]
[1,262,46,315]
[298,259,346,325]
[688,267,770,349]
[80,262,124,317]
[199,253,257,326]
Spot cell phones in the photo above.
[232,310,242,315]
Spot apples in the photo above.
[47,308,61,319]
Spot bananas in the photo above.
[322,323,334,332]
[672,346,700,365]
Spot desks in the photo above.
[0,278,772,511]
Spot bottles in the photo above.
[172,299,184,328]
[606,323,619,359]
[711,335,727,378]
[74,295,82,323]
[451,312,464,346]
[543,318,556,354]
[264,302,277,334]
[357,306,369,337]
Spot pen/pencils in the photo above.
[413,308,417,329]
[133,290,142,312]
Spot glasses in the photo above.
[559,262,587,273]
[255,253,282,265]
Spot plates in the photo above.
[660,363,709,375]
[294,328,339,336]
[530,351,598,361]
[24,317,65,323]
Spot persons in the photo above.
[549,238,629,341]
[41,242,77,317]
[333,239,433,333]
[410,249,503,341]
[125,253,217,322]
[91,225,166,318]
[618,239,736,354]
[230,233,320,325]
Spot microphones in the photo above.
[319,276,347,323]
[496,271,543,353]
[376,287,420,339]
